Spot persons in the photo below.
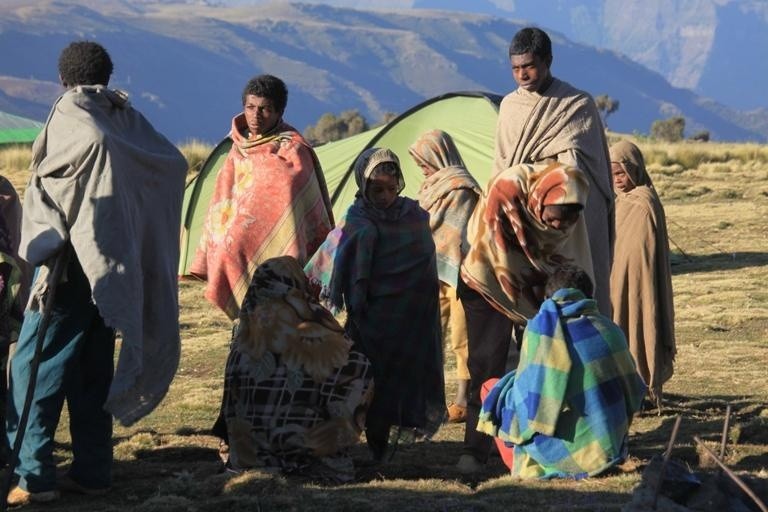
[210,254,382,488]
[0,42,191,507]
[453,159,599,479]
[185,73,338,325]
[473,263,648,482]
[609,138,680,417]
[404,127,488,426]
[317,143,452,470]
[1,173,35,474]
[493,27,616,325]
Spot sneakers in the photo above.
[5,470,114,506]
[440,453,487,478]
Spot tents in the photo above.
[179,90,504,280]
